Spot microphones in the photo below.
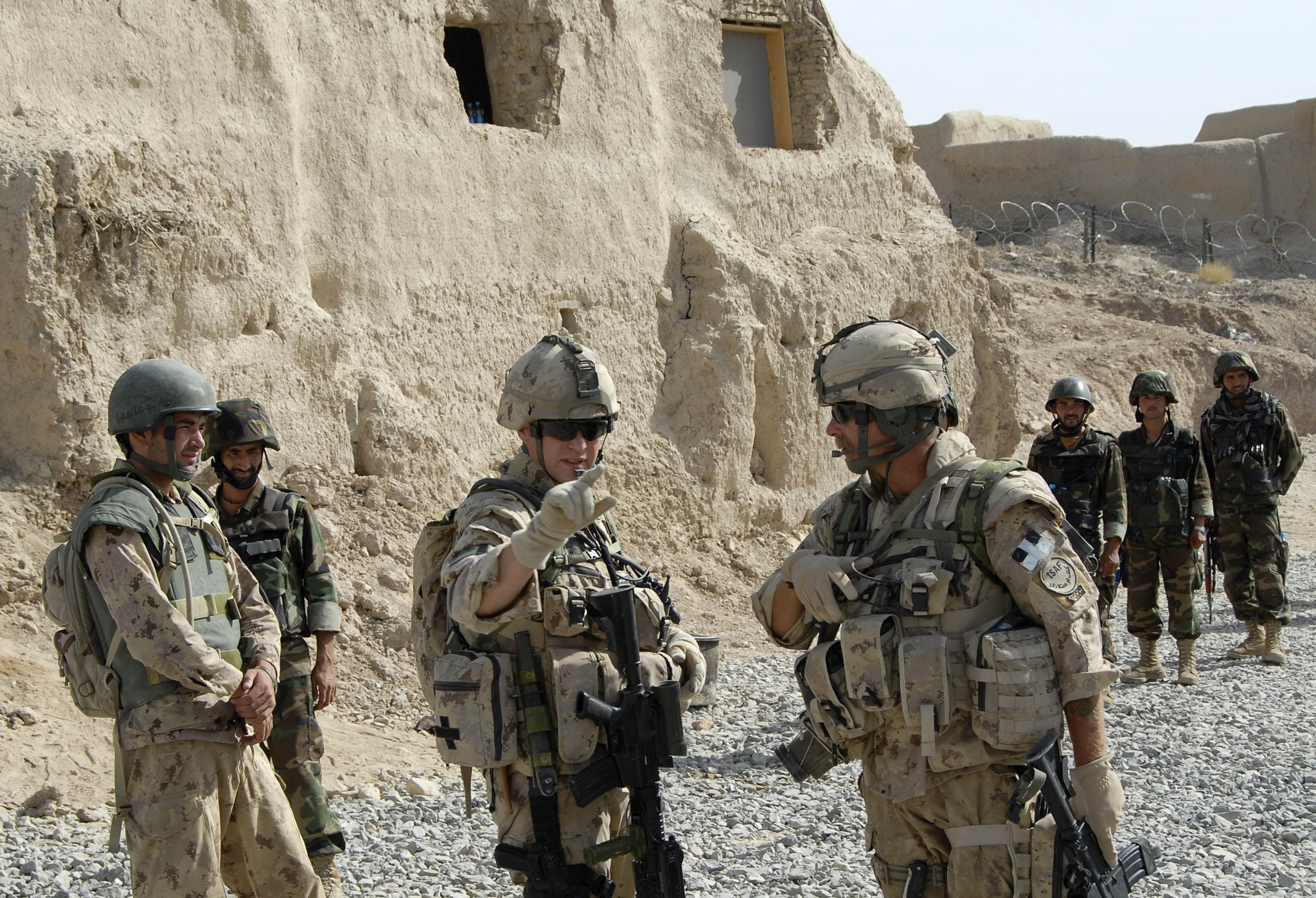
[831,440,901,458]
[573,469,587,477]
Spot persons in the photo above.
[1201,350,1305,662]
[1117,371,1214,684]
[201,397,345,897]
[441,336,707,898]
[750,318,1125,898]
[1027,378,1127,662]
[66,359,323,898]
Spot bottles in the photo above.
[1280,532,1287,542]
[467,103,475,123]
[475,102,484,123]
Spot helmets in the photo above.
[201,398,280,461]
[108,359,222,436]
[1129,370,1179,405]
[1045,378,1095,414]
[811,320,952,409]
[1213,350,1260,389]
[496,335,621,431]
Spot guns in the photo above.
[575,583,686,898]
[1023,732,1162,898]
[1203,518,1216,624]
[494,843,555,893]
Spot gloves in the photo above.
[778,549,874,622]
[511,464,615,570]
[1068,751,1124,867]
[666,629,705,715]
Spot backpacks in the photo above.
[41,479,231,718]
[412,486,538,712]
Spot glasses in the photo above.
[831,402,868,423]
[537,418,612,442]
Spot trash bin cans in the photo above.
[687,632,722,706]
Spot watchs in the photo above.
[1193,526,1209,535]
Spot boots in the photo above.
[1120,637,1166,683]
[1176,637,1198,685]
[1262,620,1286,664]
[1227,621,1266,658]
[1101,685,1115,709]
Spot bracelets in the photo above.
[255,666,277,694]
